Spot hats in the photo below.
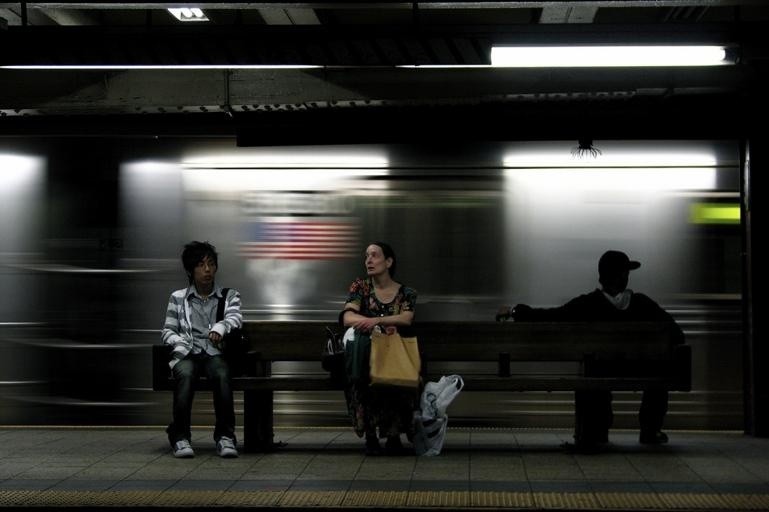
[598,250,641,273]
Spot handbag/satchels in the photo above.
[368,328,423,389]
[221,326,263,376]
[321,325,349,375]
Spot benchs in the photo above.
[149,319,695,458]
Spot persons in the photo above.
[343,240,418,450]
[496,250,683,444]
[161,239,243,457]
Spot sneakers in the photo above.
[216,436,240,458]
[173,439,195,458]
